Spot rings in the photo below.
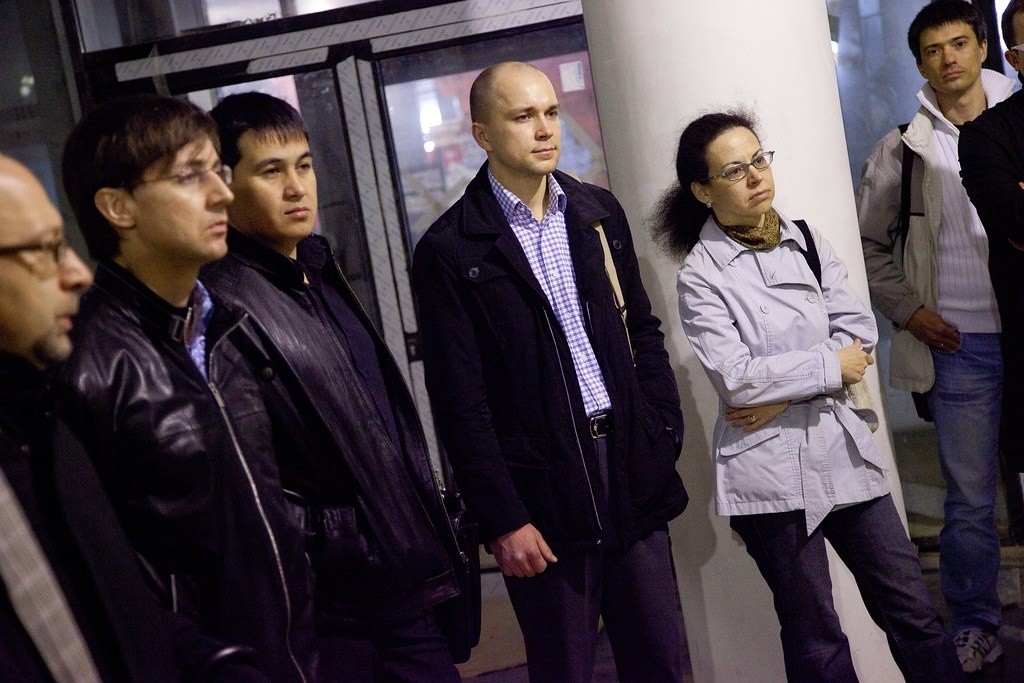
[941,344,943,347]
[749,415,755,423]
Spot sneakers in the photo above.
[953,628,1002,672]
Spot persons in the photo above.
[858,0,1017,673]
[0,89,471,683]
[643,111,968,683]
[411,61,692,683]
[958,0,1024,544]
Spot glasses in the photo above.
[0,237,68,264]
[118,164,232,189]
[1011,44,1024,51]
[708,151,775,181]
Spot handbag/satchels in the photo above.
[425,485,481,665]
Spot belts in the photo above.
[588,413,614,439]
